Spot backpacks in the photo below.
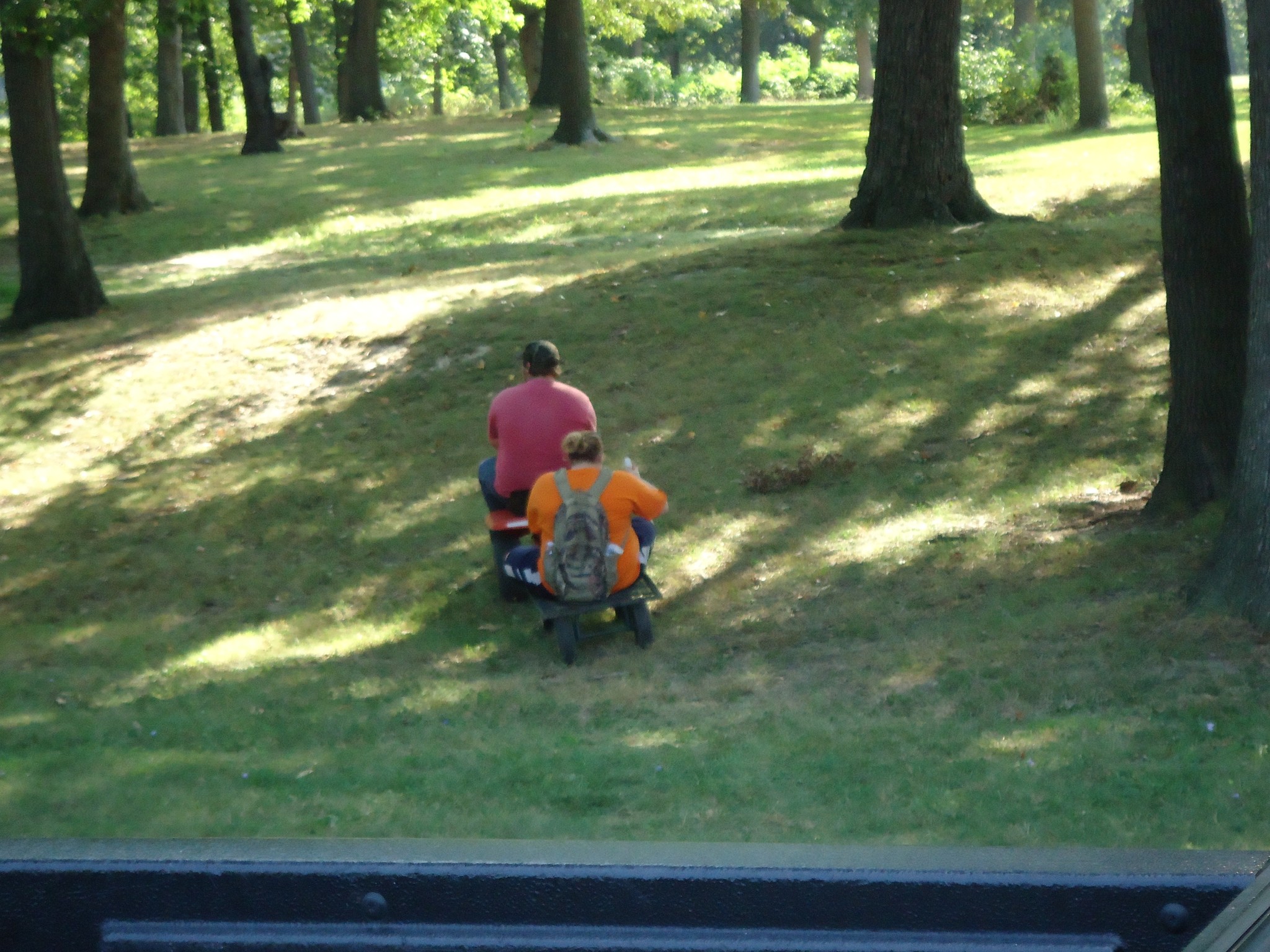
[550,467,615,603]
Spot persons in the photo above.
[479,338,597,604]
[503,431,670,604]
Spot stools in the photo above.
[486,508,532,596]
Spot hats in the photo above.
[562,431,602,456]
[516,340,561,376]
